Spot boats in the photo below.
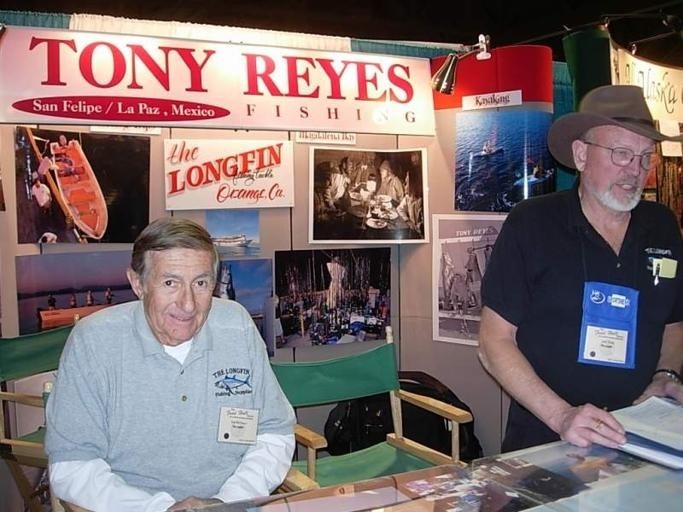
[48,141,108,242]
[473,146,503,158]
[512,168,554,188]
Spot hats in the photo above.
[380,161,393,173]
[315,161,341,174]
[547,85,683,170]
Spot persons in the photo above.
[44,216,297,512]
[314,151,424,238]
[30,154,79,243]
[477,84,683,454]
[46,288,113,311]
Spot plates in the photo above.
[365,218,387,229]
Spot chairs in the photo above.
[267,325,473,494]
[2,311,80,511]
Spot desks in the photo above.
[175,441,681,511]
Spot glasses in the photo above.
[583,140,661,171]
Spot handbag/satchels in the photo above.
[324,381,449,456]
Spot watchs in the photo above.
[652,369,681,381]
